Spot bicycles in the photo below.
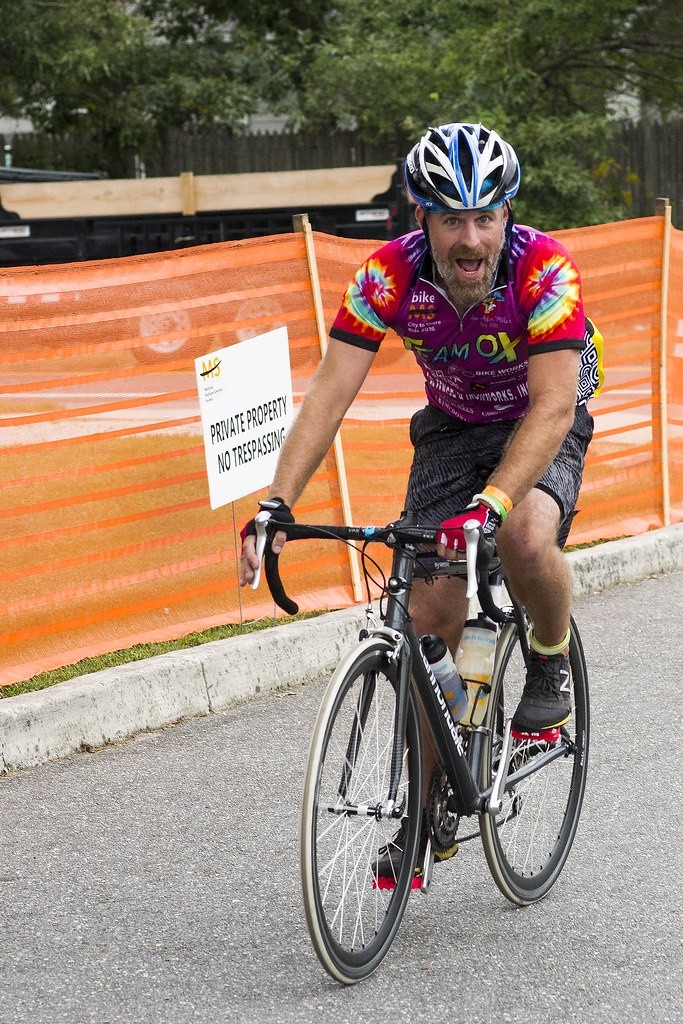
[250,498,590,987]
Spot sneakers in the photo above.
[371,806,458,878]
[511,645,573,731]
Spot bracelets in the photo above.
[473,486,513,522]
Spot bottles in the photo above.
[419,633,468,722]
[454,612,497,726]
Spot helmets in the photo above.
[403,122,521,213]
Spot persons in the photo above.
[236,121,607,882]
